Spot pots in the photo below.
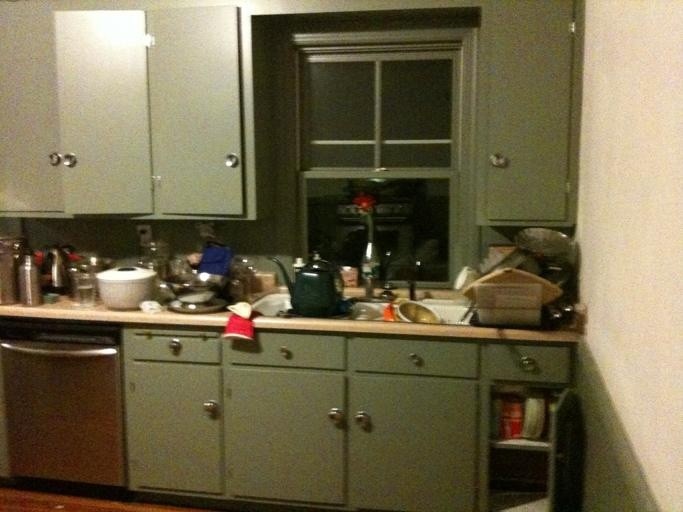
[94,265,158,312]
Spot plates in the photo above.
[167,298,235,316]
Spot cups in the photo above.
[72,272,99,310]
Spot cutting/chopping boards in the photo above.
[473,282,542,323]
[462,267,564,307]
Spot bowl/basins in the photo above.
[395,300,443,327]
[162,272,226,309]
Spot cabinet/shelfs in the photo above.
[482,339,578,507]
[227,332,479,512]
[0,323,127,498]
[477,4,577,228]
[124,319,221,502]
[2,3,257,225]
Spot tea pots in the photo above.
[272,256,346,318]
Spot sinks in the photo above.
[250,292,476,325]
